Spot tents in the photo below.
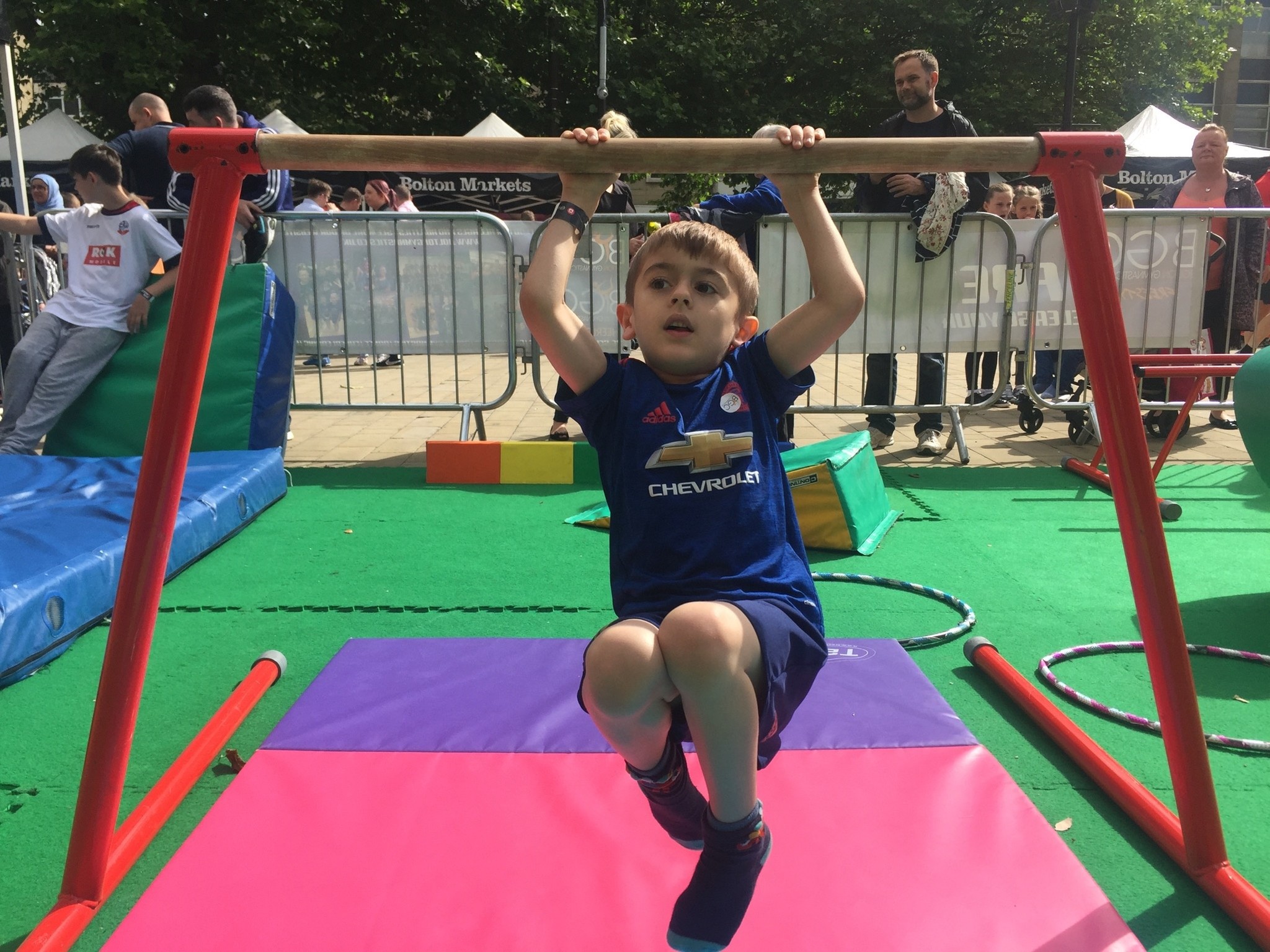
[1000,106,1269,222]
[1,109,103,165]
[390,110,606,227]
[250,106,372,205]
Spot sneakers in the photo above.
[915,428,944,455]
[242,215,277,263]
[865,427,894,449]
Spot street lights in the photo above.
[1210,47,1238,124]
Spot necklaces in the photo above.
[1191,171,1226,192]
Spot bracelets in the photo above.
[139,289,155,303]
[549,200,590,239]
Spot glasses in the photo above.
[320,194,330,199]
[30,185,47,189]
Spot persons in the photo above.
[695,125,800,446]
[519,209,537,222]
[166,83,301,441]
[287,169,447,371]
[844,50,991,448]
[0,142,193,453]
[509,105,869,952]
[95,92,188,276]
[973,124,1269,440]
[548,111,649,440]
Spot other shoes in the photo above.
[370,354,404,369]
[964,393,1012,409]
[302,356,317,365]
[1209,413,1239,430]
[549,425,570,441]
[354,355,368,365]
[1002,384,1018,402]
[315,357,330,366]
[377,354,384,362]
[1013,386,1034,402]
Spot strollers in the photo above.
[1017,228,1227,445]
